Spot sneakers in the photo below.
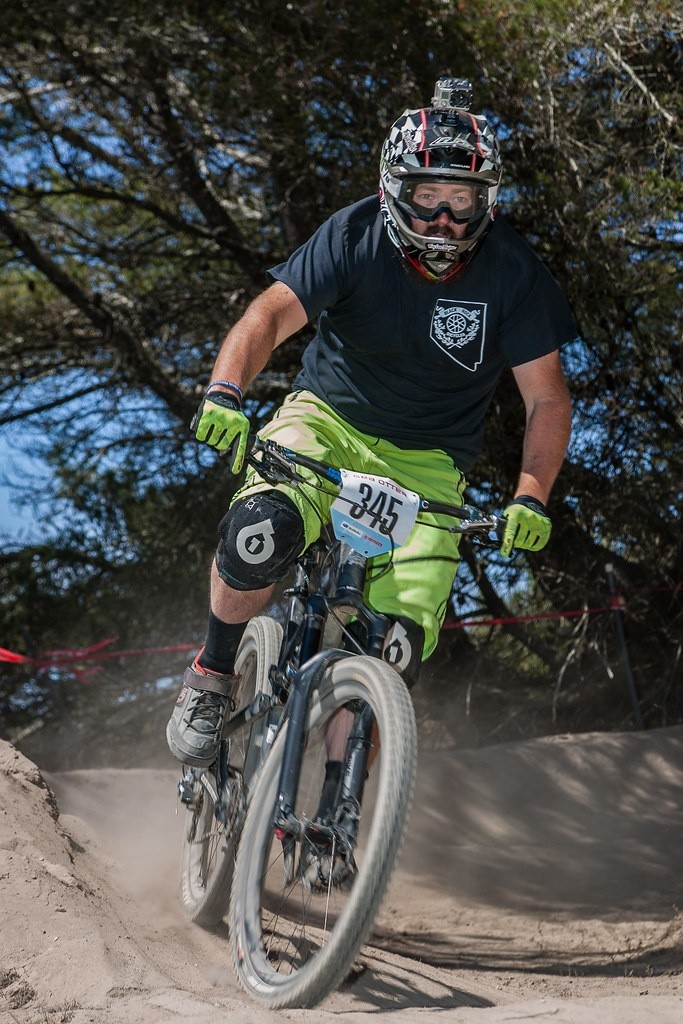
[166,646,241,769]
[296,841,349,892]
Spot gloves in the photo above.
[194,390,250,475]
[499,495,552,556]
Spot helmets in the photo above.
[380,106,504,283]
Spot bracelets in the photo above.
[204,381,245,401]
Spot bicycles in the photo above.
[169,429,508,1009]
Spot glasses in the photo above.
[396,179,488,220]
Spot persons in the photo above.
[165,77,579,895]
